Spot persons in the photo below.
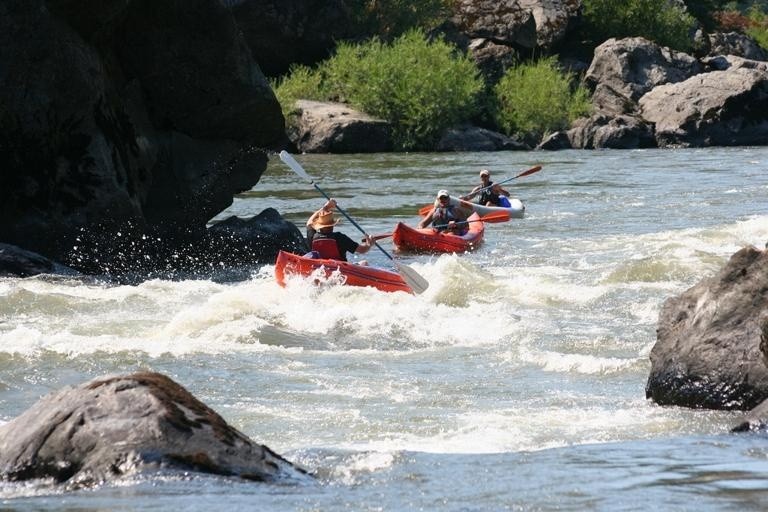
[306,198,376,267]
[418,189,467,236]
[459,170,510,207]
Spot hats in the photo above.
[310,211,342,230]
[438,190,448,199]
[480,170,488,176]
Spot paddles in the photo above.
[419,166,541,216]
[362,210,511,242]
[279,150,429,294]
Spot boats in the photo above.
[449,195,525,218]
[275,250,413,294]
[394,211,483,253]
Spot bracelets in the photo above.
[322,206,329,211]
